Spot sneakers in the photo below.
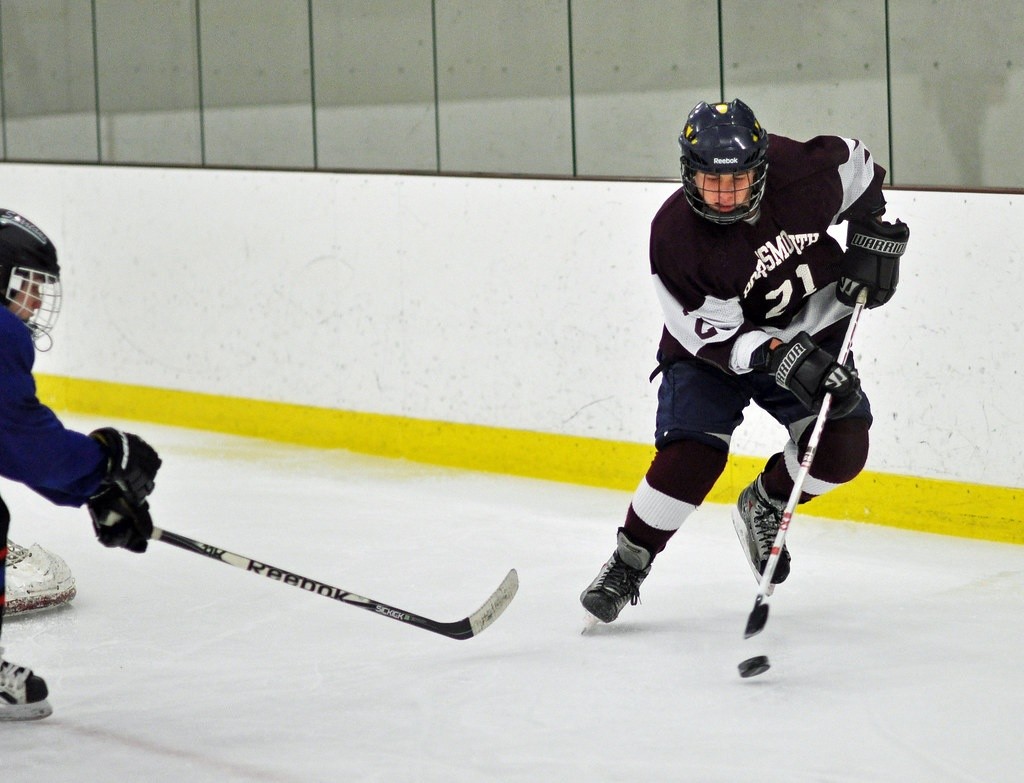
[0,657,52,720]
[731,452,791,596]
[580,527,652,637]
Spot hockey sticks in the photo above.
[151,525,520,640]
[743,287,868,639]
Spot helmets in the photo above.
[679,99,769,226]
[0,208,62,338]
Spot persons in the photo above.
[0,202,163,723]
[579,97,911,625]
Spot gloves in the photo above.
[87,482,153,553]
[768,331,861,420]
[836,208,910,308]
[90,428,162,507]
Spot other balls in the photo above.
[737,655,771,678]
